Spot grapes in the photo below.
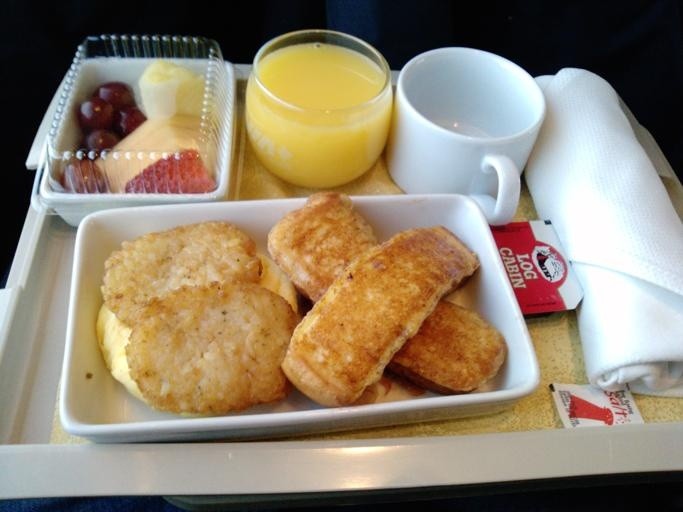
[60,80,147,194]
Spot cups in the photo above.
[384,44,548,226]
[242,25,394,192]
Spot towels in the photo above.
[524,67,683,397]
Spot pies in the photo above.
[96,191,508,418]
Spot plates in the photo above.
[57,190,544,445]
